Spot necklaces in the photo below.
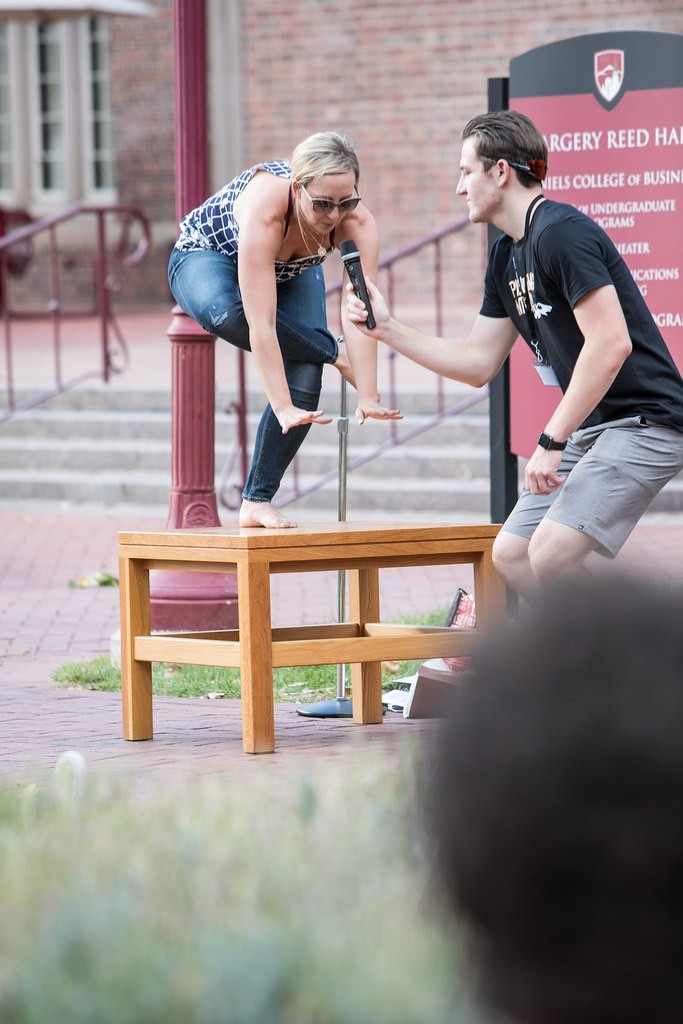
[302,224,327,257]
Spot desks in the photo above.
[118,521,505,753]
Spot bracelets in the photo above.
[537,432,568,451]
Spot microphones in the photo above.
[339,239,376,329]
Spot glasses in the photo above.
[299,184,362,213]
[481,154,548,181]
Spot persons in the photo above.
[417,568,682,1024]
[345,110,682,610]
[168,131,405,529]
